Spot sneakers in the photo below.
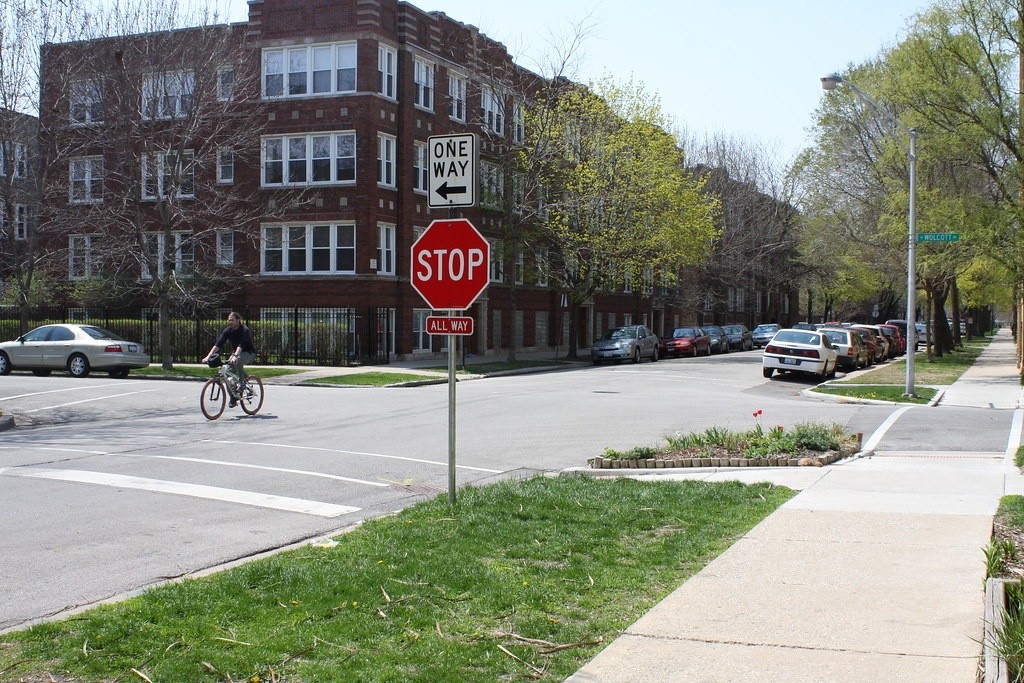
[229,397,236,407]
[236,383,247,391]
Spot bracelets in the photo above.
[233,354,239,357]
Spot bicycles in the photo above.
[204,360,263,418]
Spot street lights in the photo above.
[820,73,916,389]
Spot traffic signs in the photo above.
[427,134,476,208]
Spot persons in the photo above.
[202,312,256,408]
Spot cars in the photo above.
[661,328,710,356]
[946,319,953,330]
[885,320,919,351]
[591,325,659,366]
[703,326,730,353]
[750,324,781,348]
[824,321,842,328]
[840,323,852,328]
[724,324,754,351]
[817,328,868,374]
[914,323,934,344]
[813,322,825,329]
[762,329,839,380]
[877,325,905,357]
[791,322,817,331]
[0,325,150,378]
[847,328,883,367]
[851,324,889,362]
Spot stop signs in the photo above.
[411,220,489,310]
